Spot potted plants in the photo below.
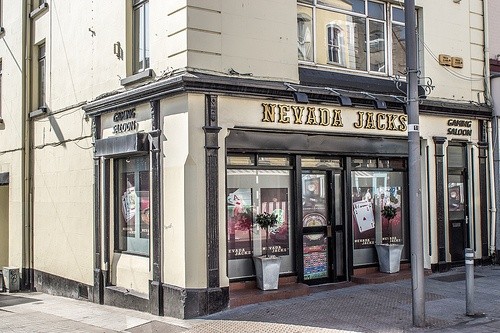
[254,211,282,290]
[375,205,404,274]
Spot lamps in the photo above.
[325,87,352,106]
[361,91,387,109]
[284,83,309,104]
